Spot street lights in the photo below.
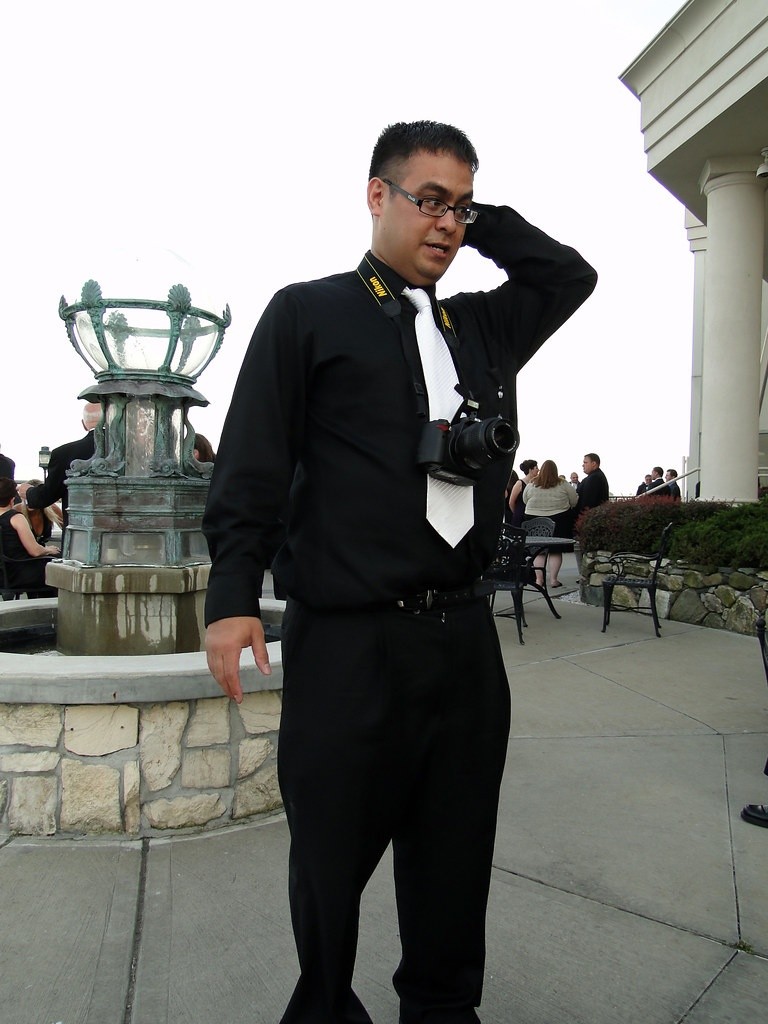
[39,446,52,483]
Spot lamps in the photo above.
[756,146,768,179]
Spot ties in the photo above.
[400,285,475,549]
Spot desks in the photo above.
[493,535,579,625]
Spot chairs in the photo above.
[599,521,674,637]
[505,516,562,619]
[484,524,530,645]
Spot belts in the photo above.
[388,581,497,610]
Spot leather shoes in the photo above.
[741,803,768,828]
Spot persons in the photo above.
[193,433,216,463]
[201,118,598,1024]
[0,402,103,600]
[636,467,700,502]
[741,759,768,830]
[508,453,610,590]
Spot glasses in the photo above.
[382,178,480,224]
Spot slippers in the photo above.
[551,582,562,588]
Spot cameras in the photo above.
[417,398,520,486]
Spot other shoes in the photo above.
[576,579,580,583]
